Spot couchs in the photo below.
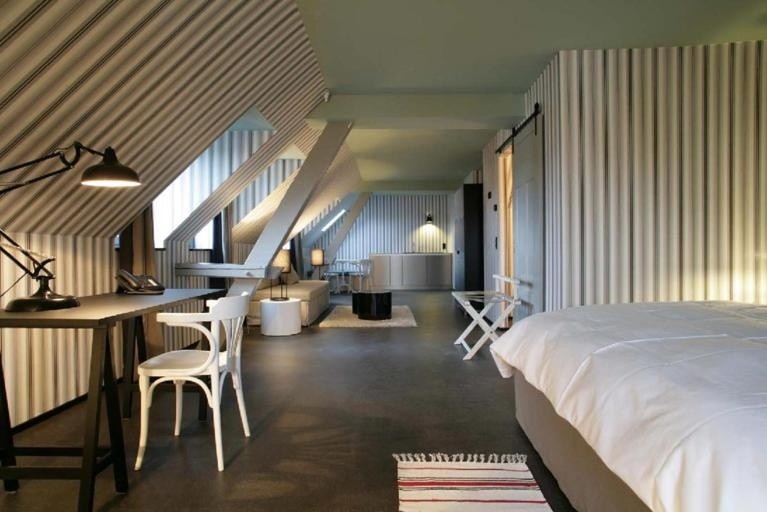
[240,264,331,328]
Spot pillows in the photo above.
[280,265,300,285]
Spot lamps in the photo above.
[0,140,142,313]
[310,248,326,281]
[267,248,292,303]
[424,213,435,227]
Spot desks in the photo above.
[260,297,302,336]
[0,285,228,512]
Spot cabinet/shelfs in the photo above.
[368,252,454,290]
[450,274,522,360]
[454,182,484,290]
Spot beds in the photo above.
[489,297,766,512]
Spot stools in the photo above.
[350,289,393,321]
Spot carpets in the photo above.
[320,303,419,331]
[393,453,554,512]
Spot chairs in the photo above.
[321,255,375,295]
[131,290,251,472]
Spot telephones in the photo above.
[116,269,165,294]
